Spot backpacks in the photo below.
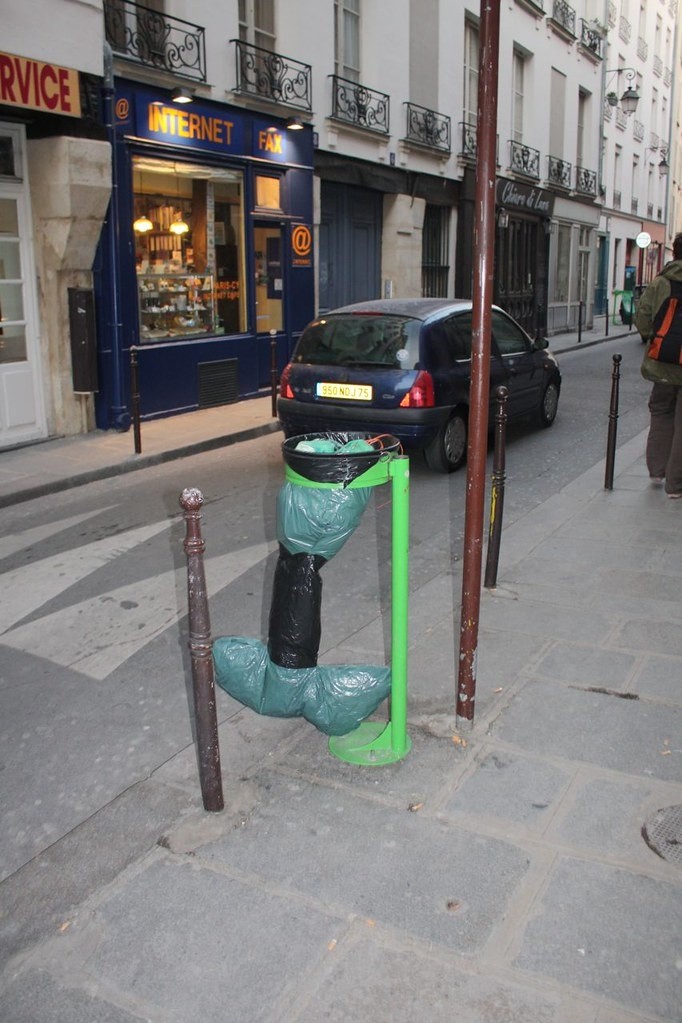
[647,281,682,365]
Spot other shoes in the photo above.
[664,488,682,498]
[649,471,666,485]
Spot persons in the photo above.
[635,232,682,498]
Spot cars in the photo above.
[277,298,562,474]
